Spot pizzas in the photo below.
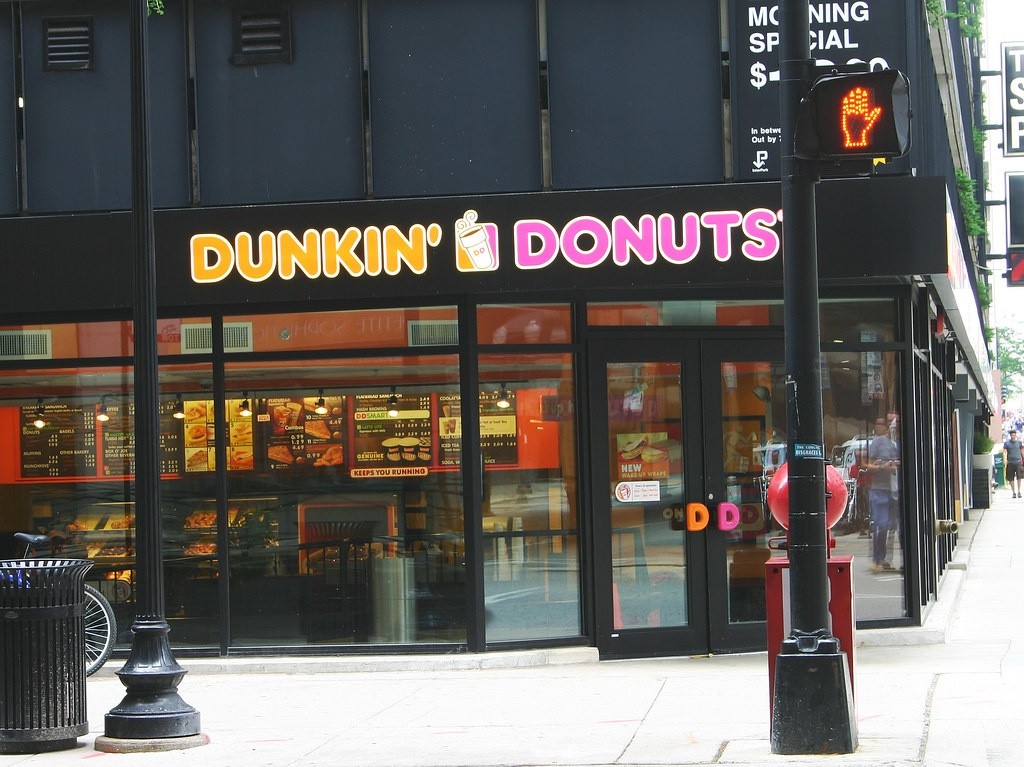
[305,420,332,439]
[268,445,294,463]
[187,450,207,467]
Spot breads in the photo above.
[66,516,136,584]
[183,512,219,577]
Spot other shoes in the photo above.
[1018,493,1022,498]
[873,560,893,572]
[1013,493,1016,498]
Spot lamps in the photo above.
[629,379,648,401]
[387,388,399,417]
[34,398,45,428]
[496,384,510,408]
[96,397,110,422]
[239,391,252,416]
[315,389,328,414]
[172,393,185,419]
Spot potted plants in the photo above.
[973,432,996,469]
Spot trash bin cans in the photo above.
[362,535,416,646]
[0,552,95,754]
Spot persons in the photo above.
[848,411,901,574]
[1003,430,1024,498]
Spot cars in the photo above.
[490,432,900,546]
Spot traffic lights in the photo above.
[802,68,914,161]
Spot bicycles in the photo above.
[13,533,117,680]
